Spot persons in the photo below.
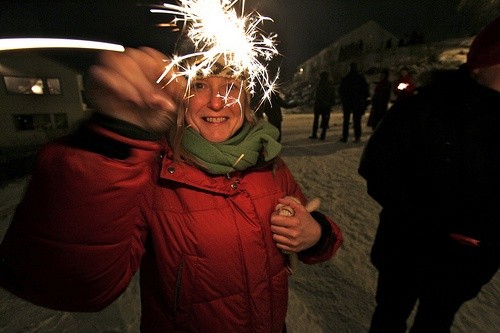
[309,70,336,141]
[260,83,303,143]
[367,67,394,132]
[358,16,500,333]
[393,67,417,95]
[337,61,370,143]
[0,31,343,333]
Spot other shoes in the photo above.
[353,139,361,142]
[309,136,317,138]
[340,138,347,142]
[319,137,324,140]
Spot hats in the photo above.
[173,22,252,94]
[467,18,500,70]
